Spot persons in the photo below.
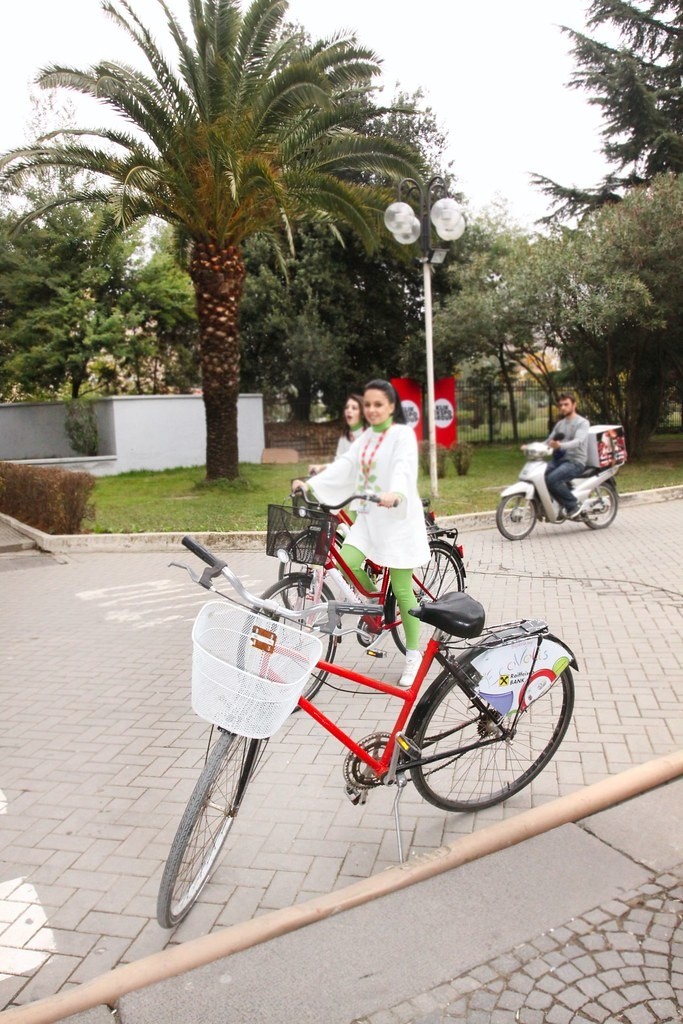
[544,393,590,519]
[291,379,432,686]
[310,394,369,476]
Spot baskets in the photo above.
[266,503,339,565]
[190,602,323,738]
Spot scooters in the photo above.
[495,432,624,541]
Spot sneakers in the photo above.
[399,654,423,686]
[365,590,381,607]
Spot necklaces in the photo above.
[361,422,394,479]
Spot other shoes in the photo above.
[568,501,584,519]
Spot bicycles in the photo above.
[155,536,580,930]
[236,486,468,714]
[279,469,442,611]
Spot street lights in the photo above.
[383,176,466,498]
